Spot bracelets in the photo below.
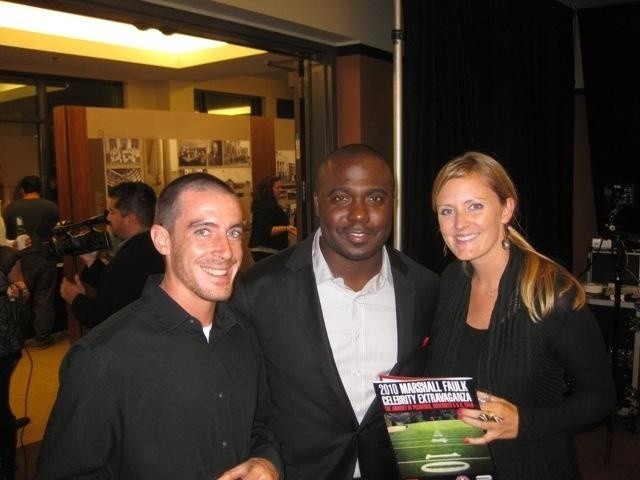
[286,226,288,231]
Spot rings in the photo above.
[478,392,502,423]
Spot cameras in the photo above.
[48,209,113,262]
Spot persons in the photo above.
[248,176,297,262]
[423,152,616,480]
[229,144,440,480]
[209,143,220,165]
[35,173,285,480]
[0,177,166,479]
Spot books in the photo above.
[373,374,502,480]
